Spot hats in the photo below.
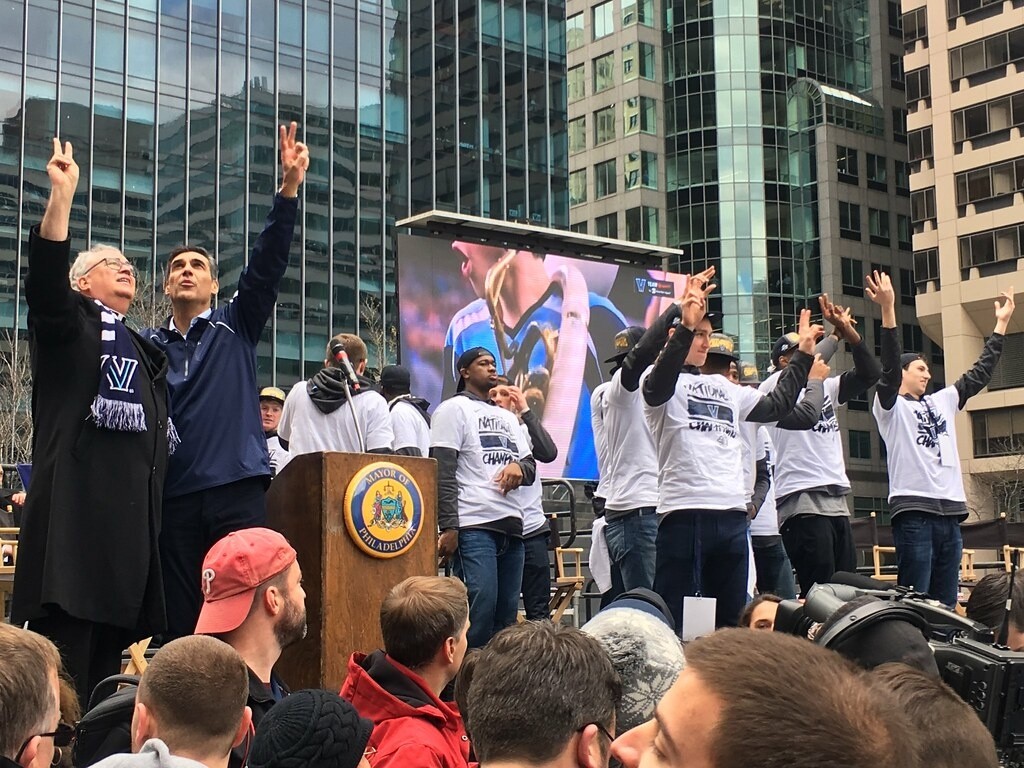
[497,375,515,386]
[597,586,675,630]
[707,333,739,360]
[457,347,495,393]
[671,311,724,327]
[259,386,286,406]
[380,365,410,396]
[194,528,297,634]
[772,332,799,362]
[900,353,918,367]
[603,326,647,363]
[245,688,372,768]
[578,608,685,733]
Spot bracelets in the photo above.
[674,300,682,314]
[519,408,530,417]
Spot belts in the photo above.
[606,506,656,525]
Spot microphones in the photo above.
[830,567,896,600]
[329,337,361,395]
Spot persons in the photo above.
[442,241,686,482]
[0,121,309,719]
[428,346,557,649]
[0,528,1024,768]
[590,266,882,630]
[865,269,1015,608]
[259,334,428,480]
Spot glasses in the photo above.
[15,722,76,763]
[578,720,623,768]
[81,258,139,278]
